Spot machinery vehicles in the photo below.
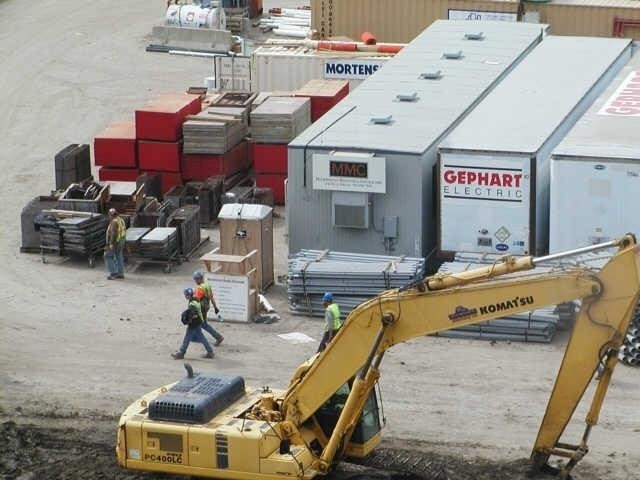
[114,230,640,480]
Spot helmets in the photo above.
[193,272,204,280]
[109,208,118,216]
[322,292,333,303]
[184,288,193,295]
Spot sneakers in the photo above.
[171,352,184,359]
[201,352,215,358]
[107,272,124,280]
[215,336,224,346]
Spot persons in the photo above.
[103,207,127,280]
[171,288,215,360]
[317,292,341,352]
[193,271,224,346]
